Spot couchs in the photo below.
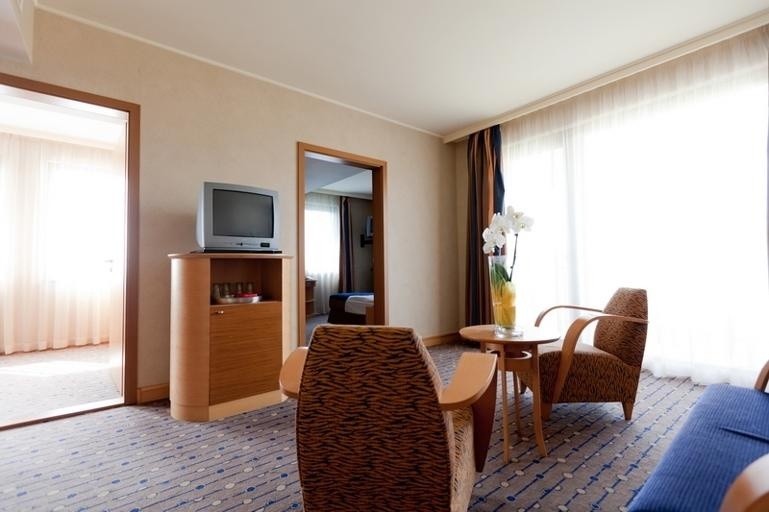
[625,359,769,511]
[519,288,648,420]
[279,323,499,512]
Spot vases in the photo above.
[487,256,523,338]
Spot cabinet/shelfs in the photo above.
[167,251,296,421]
[305,280,316,317]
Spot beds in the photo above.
[328,291,374,325]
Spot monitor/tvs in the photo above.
[195,181,283,253]
[366,216,374,237]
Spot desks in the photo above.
[459,323,561,464]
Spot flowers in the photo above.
[482,205,533,256]
